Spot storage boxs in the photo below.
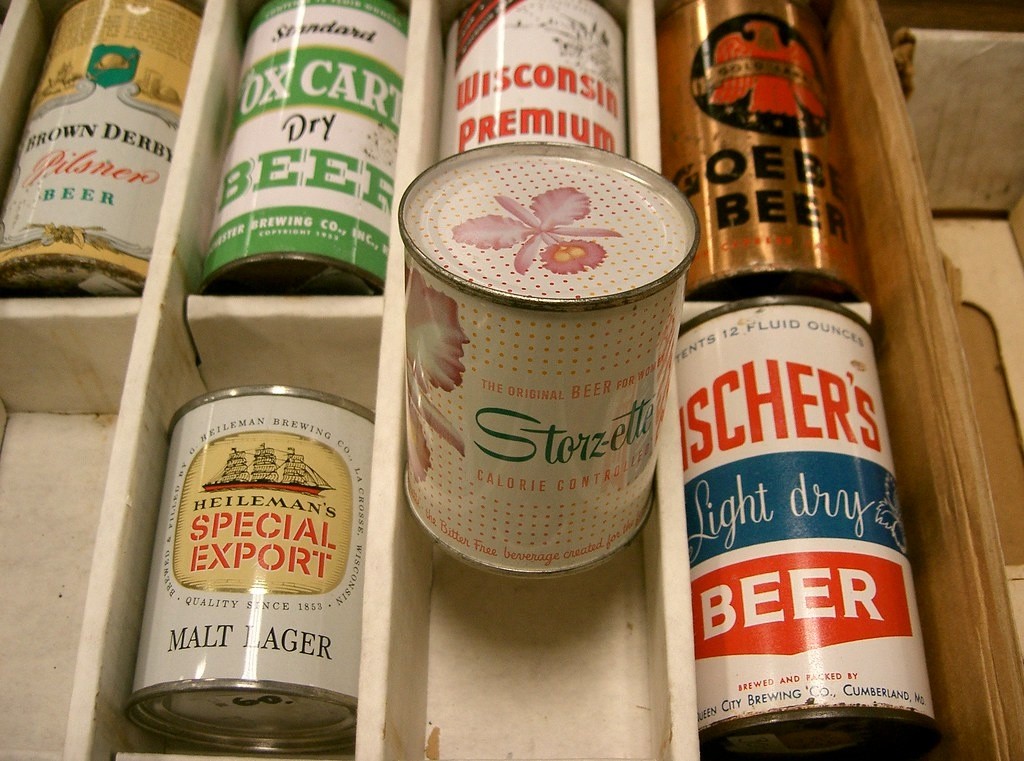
[0,0,1024,761]
